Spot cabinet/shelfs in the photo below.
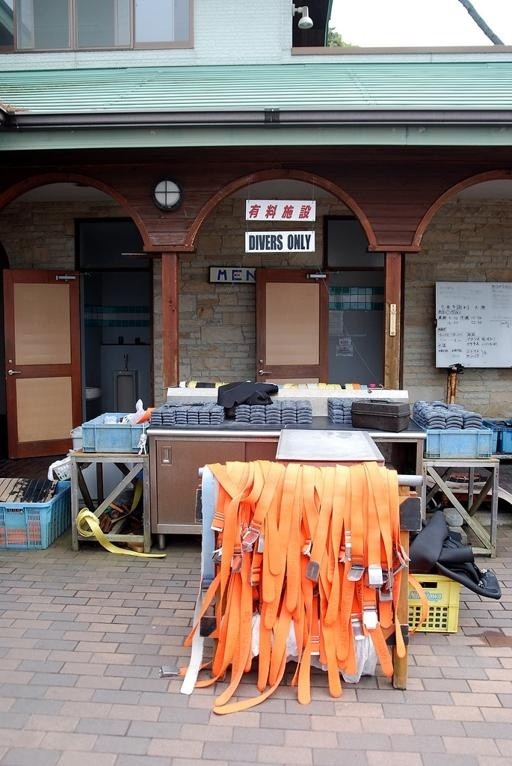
[145,433,428,551]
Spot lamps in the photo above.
[150,176,183,212]
[291,3,314,30]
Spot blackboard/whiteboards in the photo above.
[435,282,512,368]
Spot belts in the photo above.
[177,461,410,714]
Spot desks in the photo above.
[425,458,500,560]
[68,449,151,555]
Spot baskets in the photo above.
[52,458,72,480]
